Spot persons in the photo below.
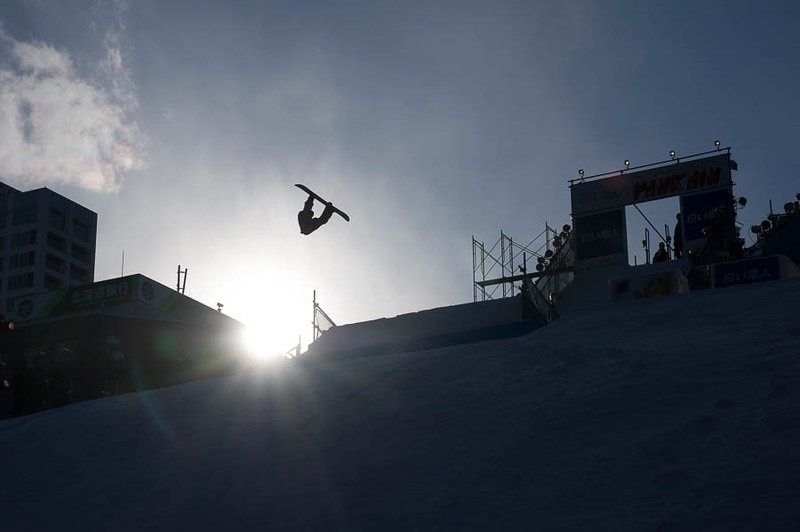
[674,213,685,259]
[652,242,668,264]
[298,195,334,235]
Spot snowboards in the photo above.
[294,183,350,221]
